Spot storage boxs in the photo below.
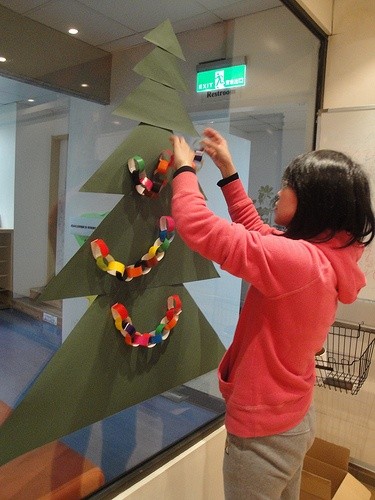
[301,436,372,500]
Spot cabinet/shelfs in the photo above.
[0,228,14,311]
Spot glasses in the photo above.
[281,180,296,189]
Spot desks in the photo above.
[0,400,105,500]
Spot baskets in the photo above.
[313,320,375,395]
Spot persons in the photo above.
[169,128,375,500]
